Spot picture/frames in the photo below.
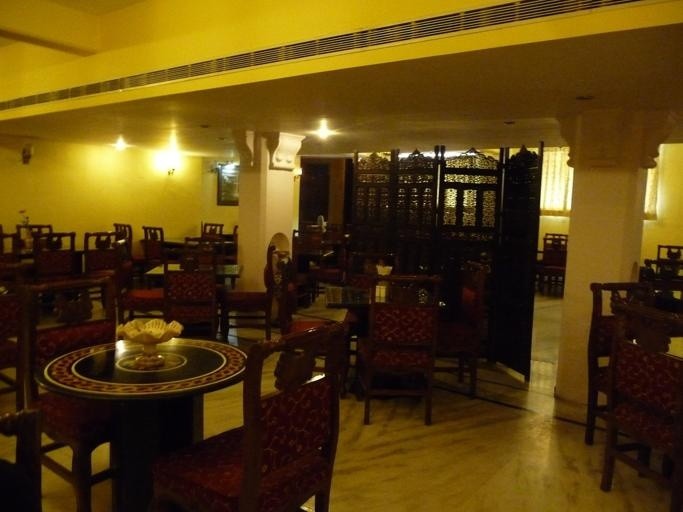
[217,163,238,207]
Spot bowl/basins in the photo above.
[375,265,394,276]
